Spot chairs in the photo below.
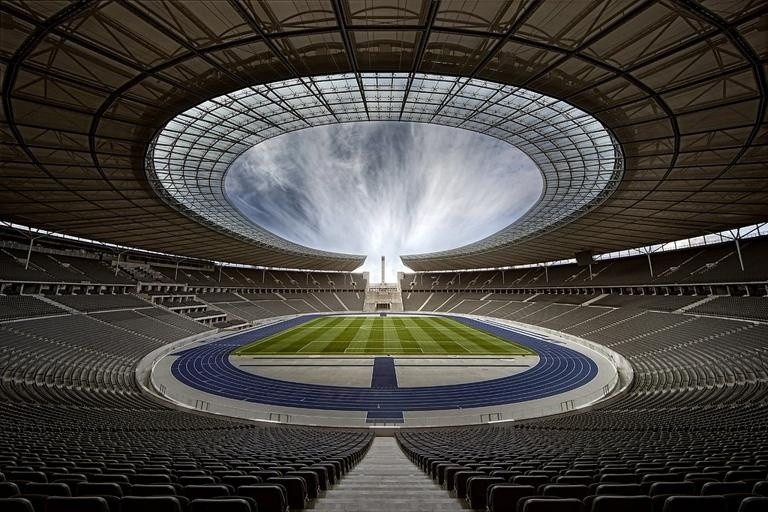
[399,265,547,328]
[548,241,767,388]
[167,253,367,344]
[167,408,374,511]
[396,388,767,511]
[1,249,167,510]
